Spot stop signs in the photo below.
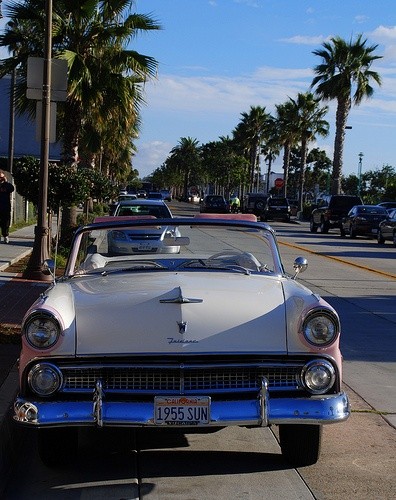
[274,178,285,189]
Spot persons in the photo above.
[0,172,15,244]
[231,195,241,213]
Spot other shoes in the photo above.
[4,236,9,244]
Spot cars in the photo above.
[107,199,182,257]
[199,195,231,215]
[376,210,396,247]
[116,190,172,202]
[375,194,396,211]
[340,204,389,239]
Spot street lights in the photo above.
[358,152,364,196]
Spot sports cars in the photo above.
[11,212,351,469]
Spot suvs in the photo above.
[260,196,291,223]
[309,194,363,234]
[240,192,272,217]
